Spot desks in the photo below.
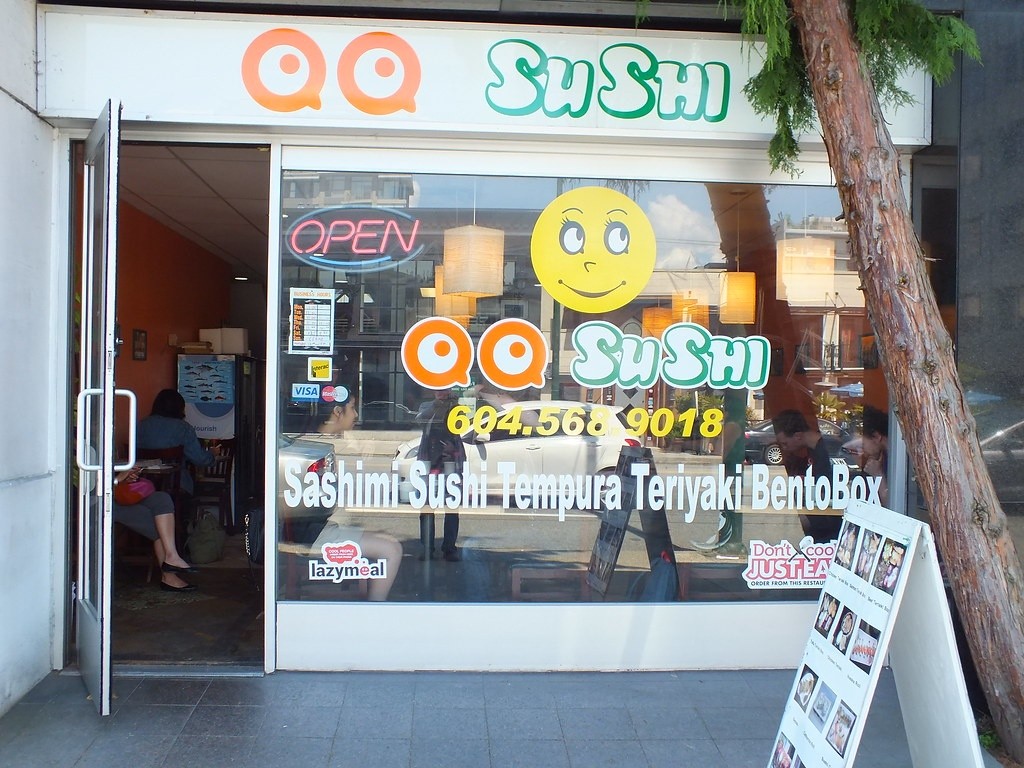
[132,464,178,492]
[734,505,844,515]
[345,504,504,602]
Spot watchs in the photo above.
[113,478,119,485]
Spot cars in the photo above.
[279,434,340,522]
[841,402,1023,509]
[740,418,853,467]
[387,400,642,518]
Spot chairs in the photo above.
[282,500,368,602]
[122,443,200,558]
[510,562,591,603]
[186,437,237,535]
[673,563,751,602]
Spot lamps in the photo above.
[642,289,710,340]
[434,182,503,328]
[776,192,835,300]
[719,193,756,324]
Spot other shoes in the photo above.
[160,582,197,592]
[443,552,458,561]
[418,553,433,561]
[161,561,200,573]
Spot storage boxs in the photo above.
[199,328,249,354]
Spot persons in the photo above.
[416,391,467,560]
[136,388,220,568]
[770,409,856,541]
[70,403,199,592]
[840,405,889,508]
[282,382,403,602]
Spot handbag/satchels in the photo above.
[244,510,263,563]
[114,477,155,506]
[187,505,225,564]
